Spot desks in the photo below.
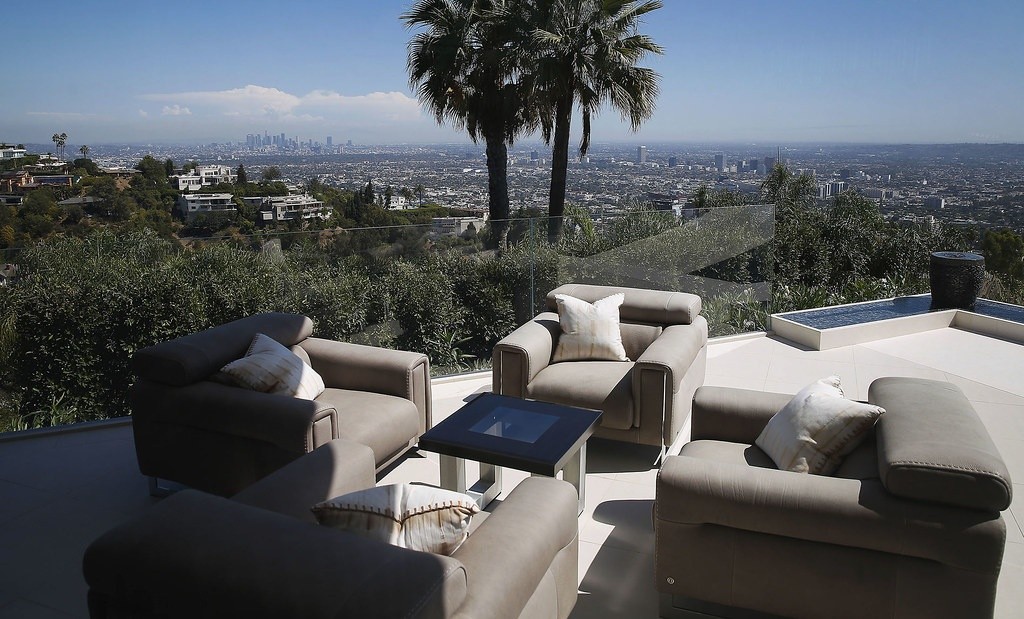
[420,393,603,519]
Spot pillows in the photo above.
[755,378,887,480]
[552,291,631,363]
[301,482,479,558]
[222,333,326,405]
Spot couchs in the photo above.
[653,373,1015,619]
[82,469,581,619]
[494,284,708,467]
[135,316,434,499]
